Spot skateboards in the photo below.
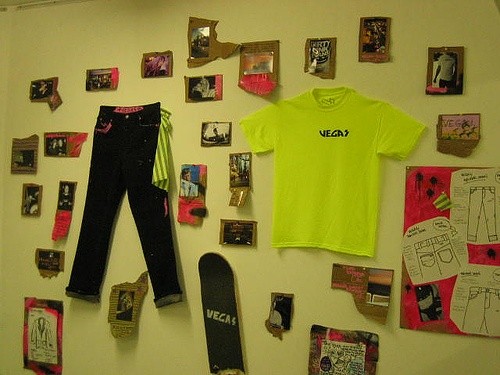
[198,253,246,375]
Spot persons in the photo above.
[47,138,73,210]
[212,127,225,142]
[181,167,191,181]
[433,51,458,87]
